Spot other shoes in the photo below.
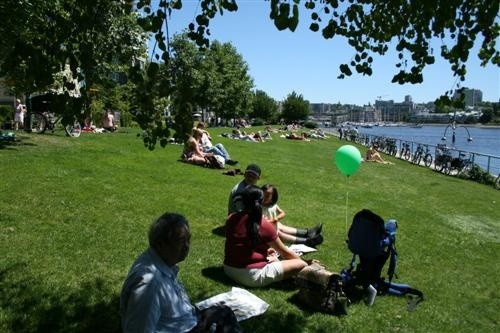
[304,223,323,246]
[226,160,238,165]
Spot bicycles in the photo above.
[30,101,81,138]
[400,143,451,175]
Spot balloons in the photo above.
[335,145,361,176]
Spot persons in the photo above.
[13,98,384,333]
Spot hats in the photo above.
[245,163,261,176]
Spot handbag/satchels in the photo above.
[204,154,225,168]
[294,265,350,316]
